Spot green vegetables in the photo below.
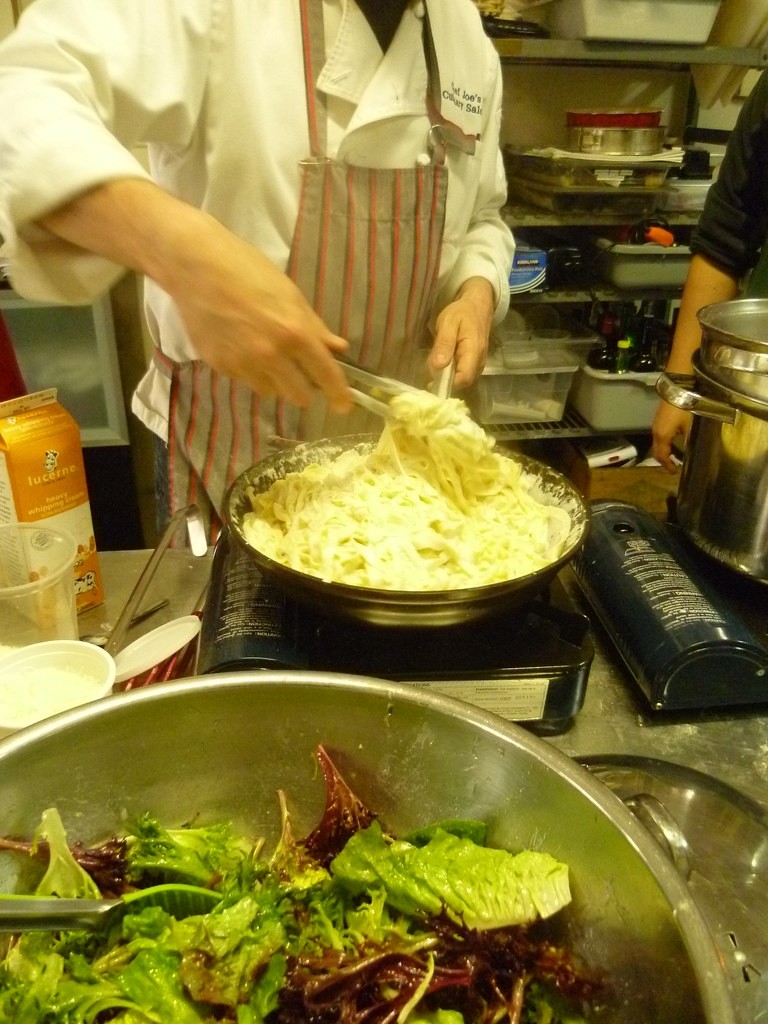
[0,741,613,1024]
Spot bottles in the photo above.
[585,314,673,375]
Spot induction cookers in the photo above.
[189,515,767,737]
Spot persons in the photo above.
[0,0,518,551]
[652,66,768,475]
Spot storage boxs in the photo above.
[560,439,637,469]
[488,315,599,371]
[570,365,663,431]
[589,234,692,290]
[518,0,723,45]
[476,363,579,424]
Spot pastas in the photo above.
[254,395,571,588]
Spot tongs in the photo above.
[332,358,487,467]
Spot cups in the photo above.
[0,523,79,657]
[0,638,117,741]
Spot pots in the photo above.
[225,434,591,627]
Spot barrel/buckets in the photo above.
[656,297,768,576]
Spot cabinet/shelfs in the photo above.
[476,39,768,441]
[0,270,159,549]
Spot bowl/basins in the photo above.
[1,669,739,1024]
